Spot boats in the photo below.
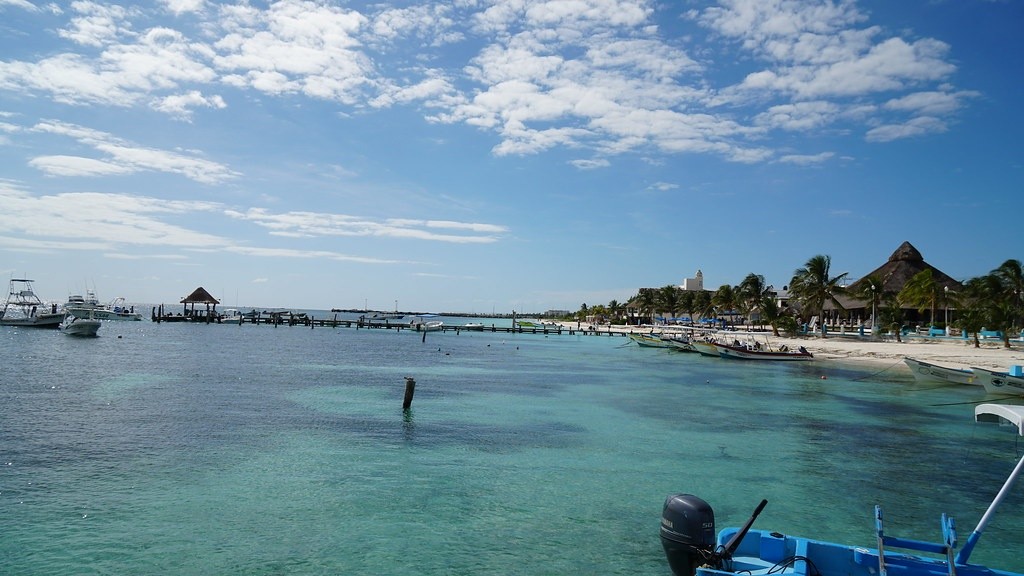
[969,365,1024,396]
[670,338,691,350]
[659,338,674,347]
[279,312,307,317]
[710,335,814,361]
[215,309,244,324]
[691,339,723,356]
[58,310,102,337]
[408,320,444,332]
[364,311,406,320]
[69,296,143,320]
[658,402,1024,576]
[263,310,289,315]
[639,334,665,347]
[903,356,981,388]
[462,322,484,332]
[0,278,70,327]
[630,336,644,346]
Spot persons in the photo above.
[578,322,580,331]
[359,317,362,322]
[361,315,365,322]
[607,321,612,330]
[593,322,599,331]
